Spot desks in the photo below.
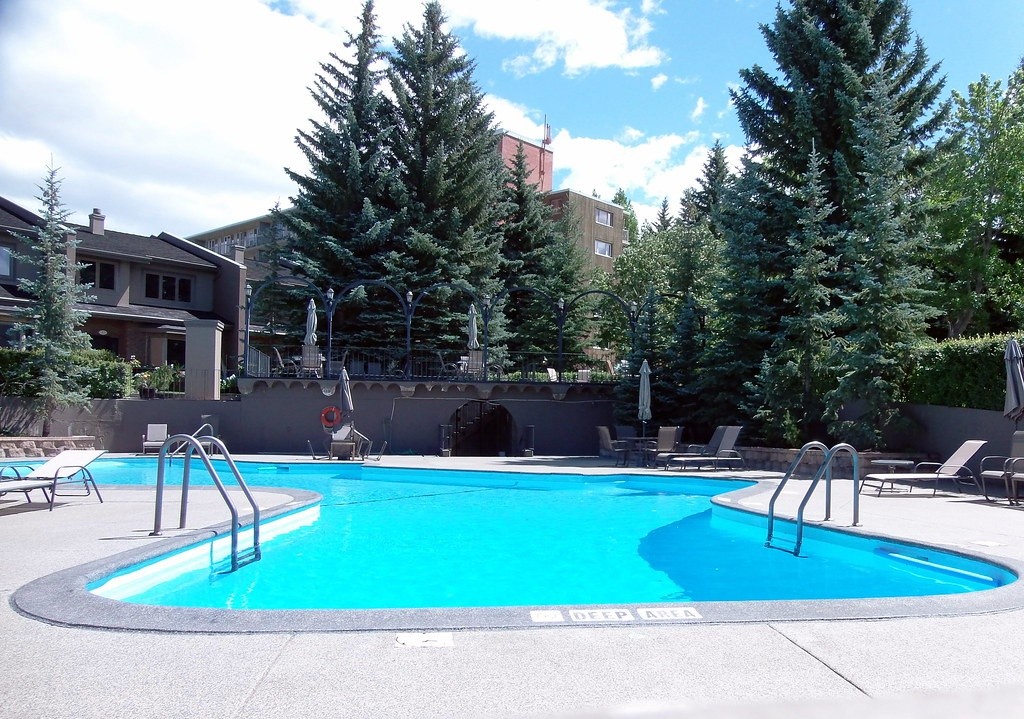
[457,361,489,364]
[618,436,659,466]
[291,355,327,362]
[869,459,915,491]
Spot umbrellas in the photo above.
[638,360,652,447]
[466,304,479,372]
[304,298,318,378]
[1002,338,1024,431]
[338,364,354,439]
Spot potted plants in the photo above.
[133,364,175,399]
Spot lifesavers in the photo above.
[320,405,342,428]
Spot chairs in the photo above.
[0,449,111,511]
[979,431,1023,505]
[142,423,171,454]
[856,439,989,499]
[598,425,750,470]
[326,349,349,379]
[189,434,223,456]
[328,420,355,461]
[546,367,566,384]
[577,369,591,383]
[437,350,484,383]
[273,347,300,378]
[605,358,640,382]
[1009,457,1024,505]
[301,344,322,379]
